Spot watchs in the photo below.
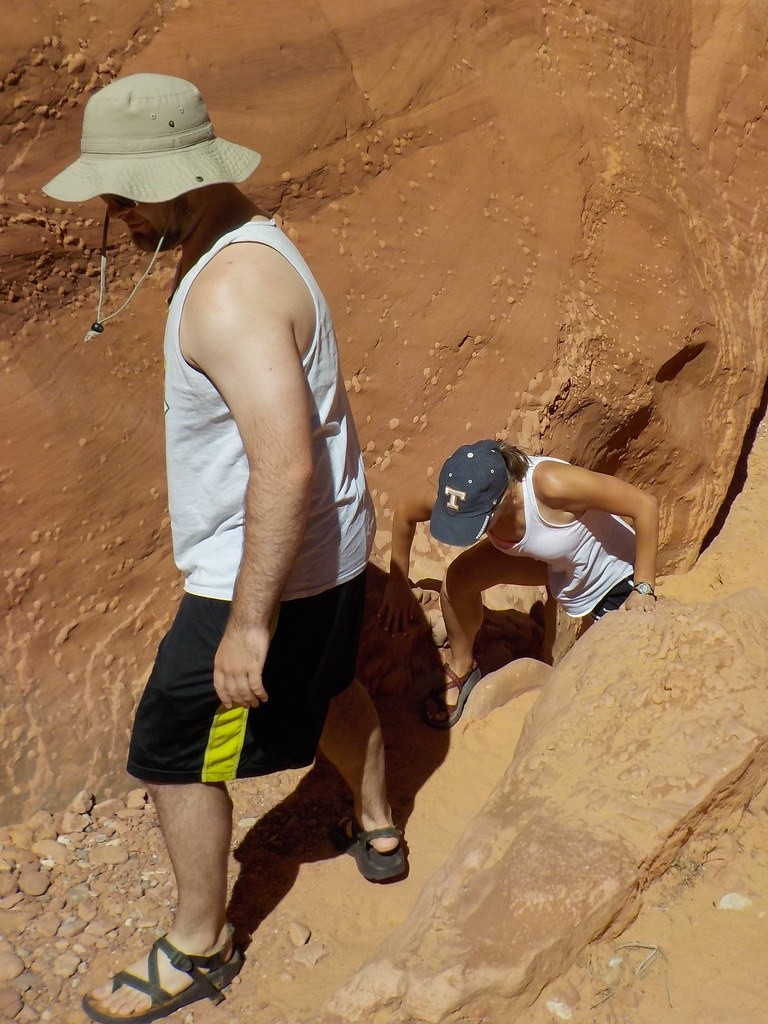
[632,582,655,596]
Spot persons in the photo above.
[41,74,412,1024]
[376,439,660,729]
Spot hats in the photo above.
[430,438,509,547]
[41,73,262,204]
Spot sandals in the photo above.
[330,809,408,882]
[419,658,481,730]
[80,923,243,1024]
